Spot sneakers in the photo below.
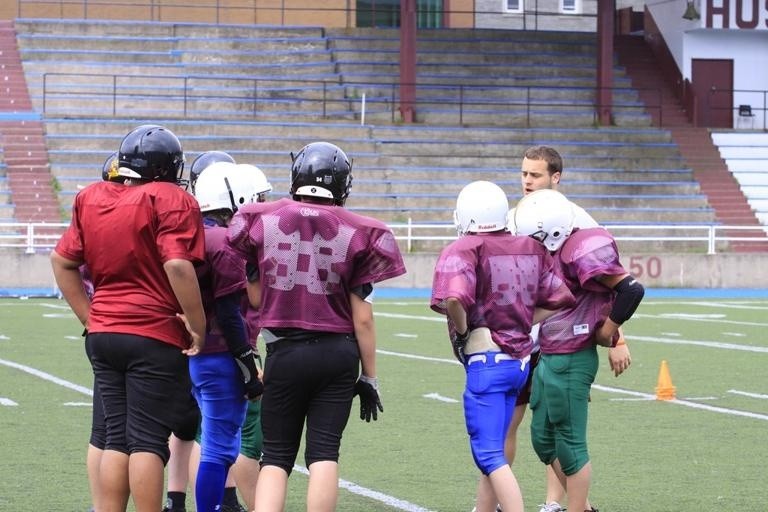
[161,505,186,512]
[539,500,561,512]
[222,501,246,512]
[584,506,599,512]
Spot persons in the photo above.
[502,143,580,511]
[49,124,207,512]
[164,148,276,512]
[429,175,576,511]
[225,142,406,512]
[512,188,645,511]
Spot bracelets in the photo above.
[616,341,626,346]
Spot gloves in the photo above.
[353,373,384,423]
[451,326,470,364]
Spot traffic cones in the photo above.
[654,360,678,400]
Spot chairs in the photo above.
[737,105,756,130]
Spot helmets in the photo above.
[102,151,131,183]
[190,151,273,214]
[117,124,190,191]
[512,189,575,252]
[290,141,354,208]
[452,181,510,238]
